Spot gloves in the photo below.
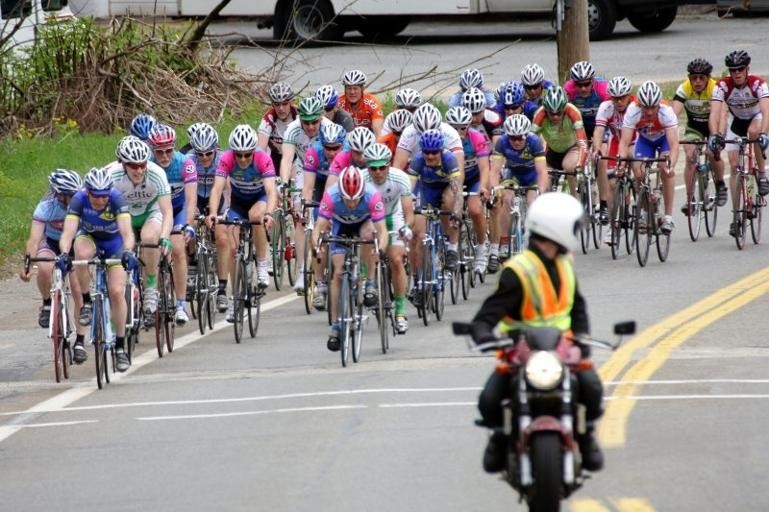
[709,135,716,150]
[759,134,769,148]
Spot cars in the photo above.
[257,1,678,41]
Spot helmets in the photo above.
[636,80,662,107]
[446,60,596,138]
[269,71,445,202]
[606,76,632,97]
[46,112,259,194]
[725,50,751,68]
[687,58,712,76]
[524,192,588,252]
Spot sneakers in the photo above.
[599,172,769,236]
[292,241,509,351]
[484,432,510,473]
[38,247,279,370]
[574,430,602,470]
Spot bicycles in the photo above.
[678,139,768,250]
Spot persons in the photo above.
[446,50,769,272]
[20,67,468,371]
[476,192,604,472]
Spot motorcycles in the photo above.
[452,320,636,512]
